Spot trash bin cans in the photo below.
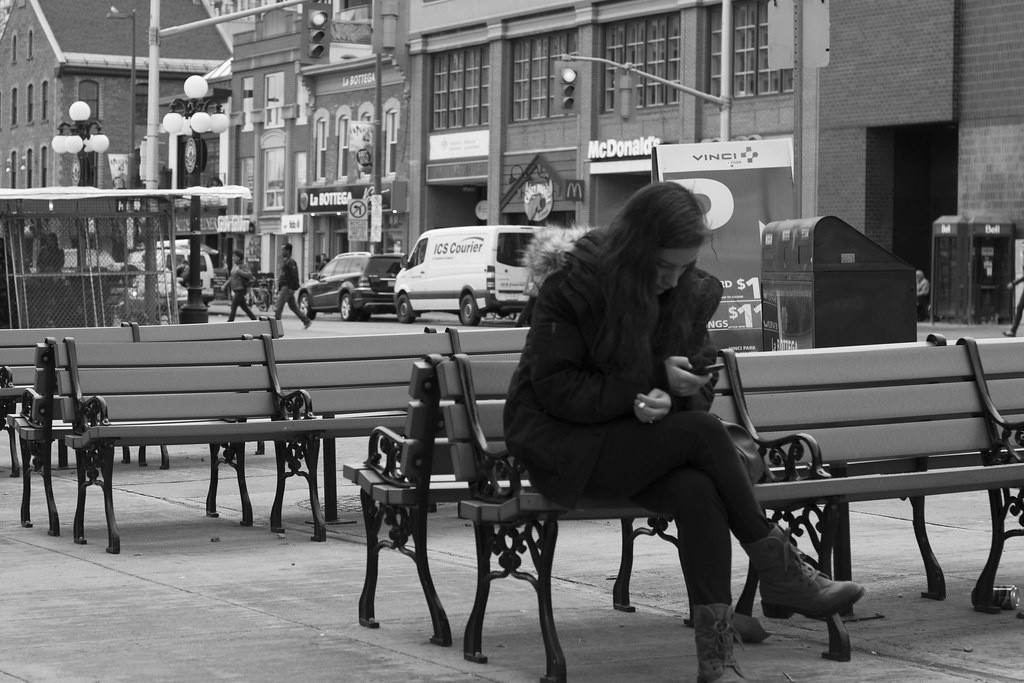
[214,269,229,300]
[760,215,918,354]
[930,213,1016,325]
[250,272,274,306]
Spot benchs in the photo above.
[0,324,1024,683]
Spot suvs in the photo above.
[296,252,405,321]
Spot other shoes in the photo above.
[305,321,312,330]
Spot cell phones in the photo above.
[691,364,726,375]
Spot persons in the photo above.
[219,250,257,322]
[273,242,312,329]
[174,259,191,289]
[37,231,65,274]
[1003,241,1024,336]
[316,253,329,271]
[916,271,930,319]
[501,179,865,683]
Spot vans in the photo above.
[122,250,215,308]
[394,225,544,325]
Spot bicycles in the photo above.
[245,278,278,311]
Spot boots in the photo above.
[691,602,745,683]
[740,521,866,620]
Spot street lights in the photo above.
[106,12,138,255]
[160,75,230,324]
[49,101,110,270]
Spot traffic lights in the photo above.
[301,2,332,64]
[561,67,576,110]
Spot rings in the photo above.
[650,416,655,423]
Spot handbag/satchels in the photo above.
[712,413,765,486]
[242,282,251,294]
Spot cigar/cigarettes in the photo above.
[637,401,646,409]
